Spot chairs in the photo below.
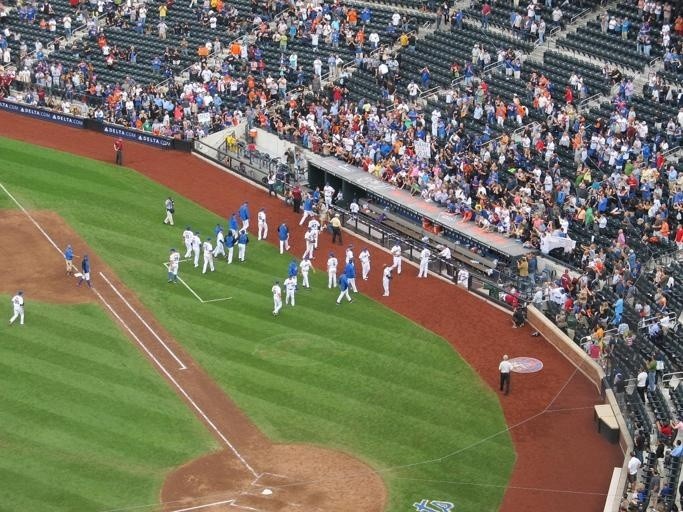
[1,0,218,106]
[548,284,683,512]
[556,152,682,284]
[216,0,683,151]
